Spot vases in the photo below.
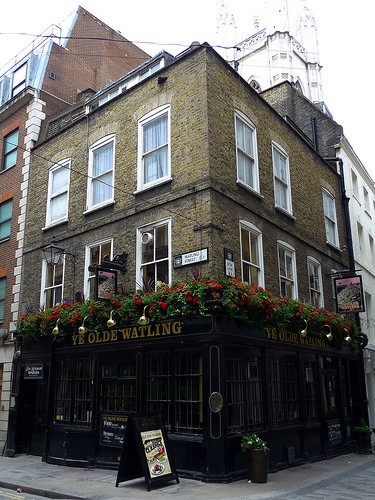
[247,448,267,484]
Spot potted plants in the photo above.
[353,426,372,453]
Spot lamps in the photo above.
[322,325,333,342]
[52,318,60,335]
[137,305,149,326]
[41,234,78,267]
[342,327,352,344]
[299,318,308,337]
[107,309,116,327]
[78,316,89,334]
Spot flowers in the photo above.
[240,432,270,452]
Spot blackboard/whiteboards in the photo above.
[114,410,181,492]
